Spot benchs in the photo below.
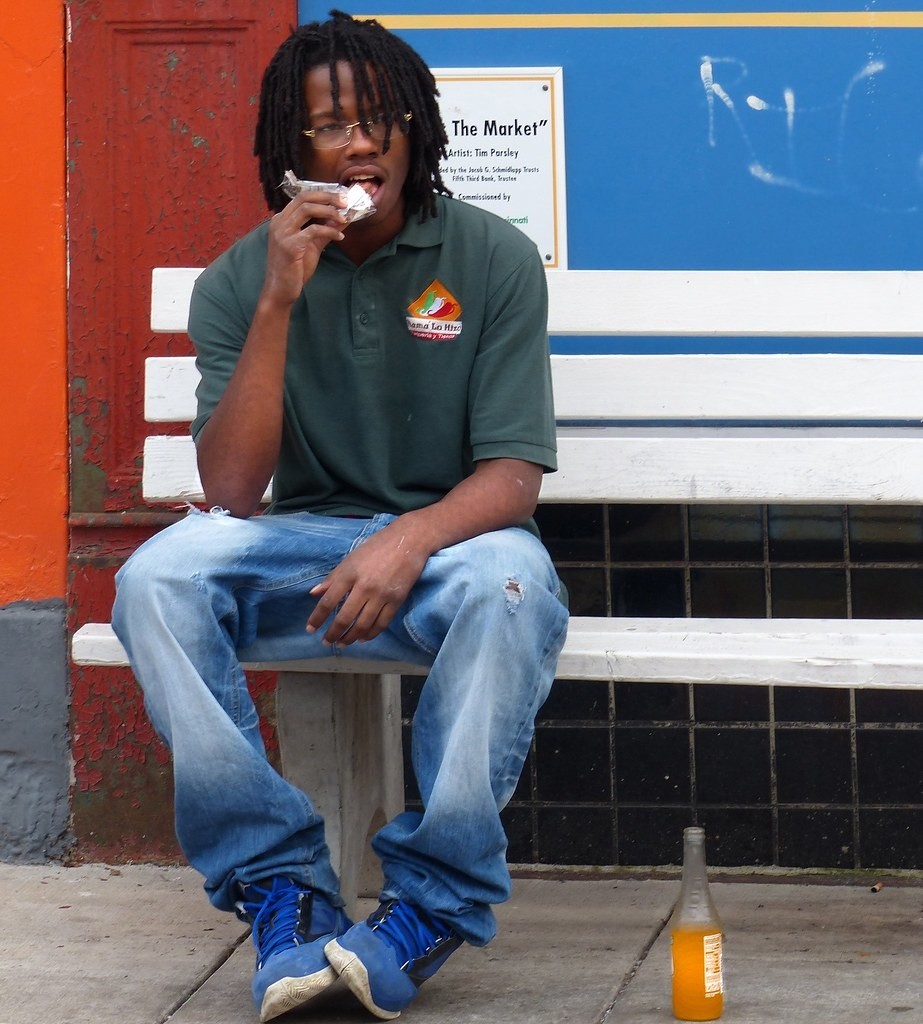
[71,266,923,926]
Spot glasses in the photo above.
[300,111,413,150]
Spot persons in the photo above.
[106,9,574,1024]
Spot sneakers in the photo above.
[323,900,465,1020]
[235,877,356,1022]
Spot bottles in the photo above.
[672,827,723,1020]
